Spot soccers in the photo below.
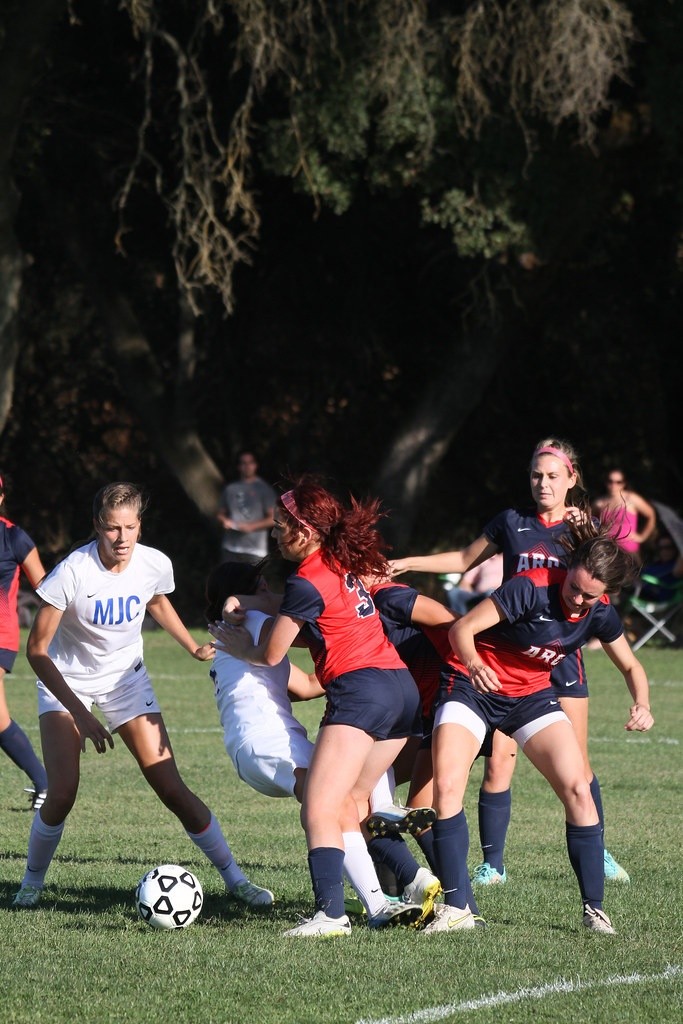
[135,865,203,931]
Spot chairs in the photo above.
[622,572,683,651]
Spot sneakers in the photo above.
[343,893,403,914]
[367,899,423,931]
[364,797,438,840]
[582,903,619,936]
[603,848,630,883]
[400,867,457,928]
[22,786,49,812]
[470,861,506,889]
[422,901,474,934]
[224,880,275,906]
[472,913,486,928]
[280,909,351,937]
[11,882,41,911]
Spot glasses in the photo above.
[608,479,623,485]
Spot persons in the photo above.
[384,438,630,884]
[217,453,275,566]
[206,483,486,938]
[424,502,654,932]
[449,550,503,611]
[0,477,48,809]
[204,561,438,929]
[15,484,274,904]
[593,470,655,553]
